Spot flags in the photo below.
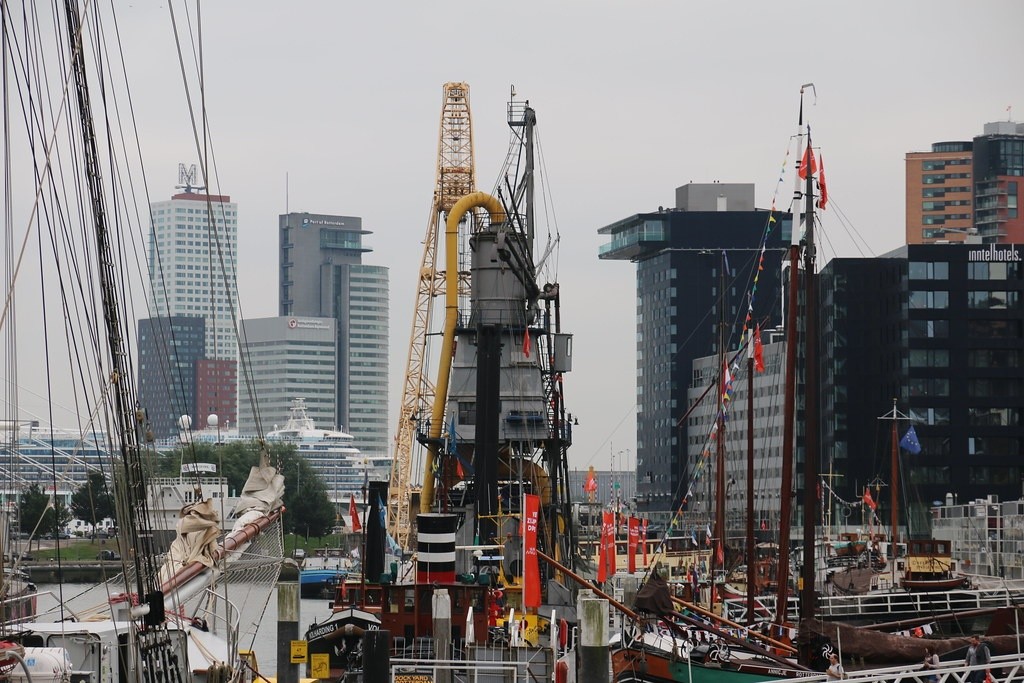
[349,496,361,533]
[687,560,706,592]
[600,512,647,581]
[902,624,934,638]
[679,609,796,655]
[754,327,765,371]
[523,330,532,357]
[864,491,876,508]
[722,358,730,400]
[430,415,465,480]
[706,526,712,545]
[819,158,828,209]
[378,497,386,528]
[900,427,921,453]
[523,493,541,607]
[691,532,697,546]
[799,146,817,179]
[717,544,723,564]
[585,474,597,491]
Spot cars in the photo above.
[86,530,111,539]
[13,551,35,561]
[11,529,40,540]
[293,548,304,558]
[43,531,70,539]
[96,549,121,559]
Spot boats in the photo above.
[299,85,1024,683]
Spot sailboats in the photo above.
[0,0,286,683]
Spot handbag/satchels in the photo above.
[989,672,998,683]
[986,673,991,683]
[837,665,848,680]
[919,665,930,679]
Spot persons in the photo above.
[923,645,941,683]
[965,635,1002,683]
[826,653,844,682]
